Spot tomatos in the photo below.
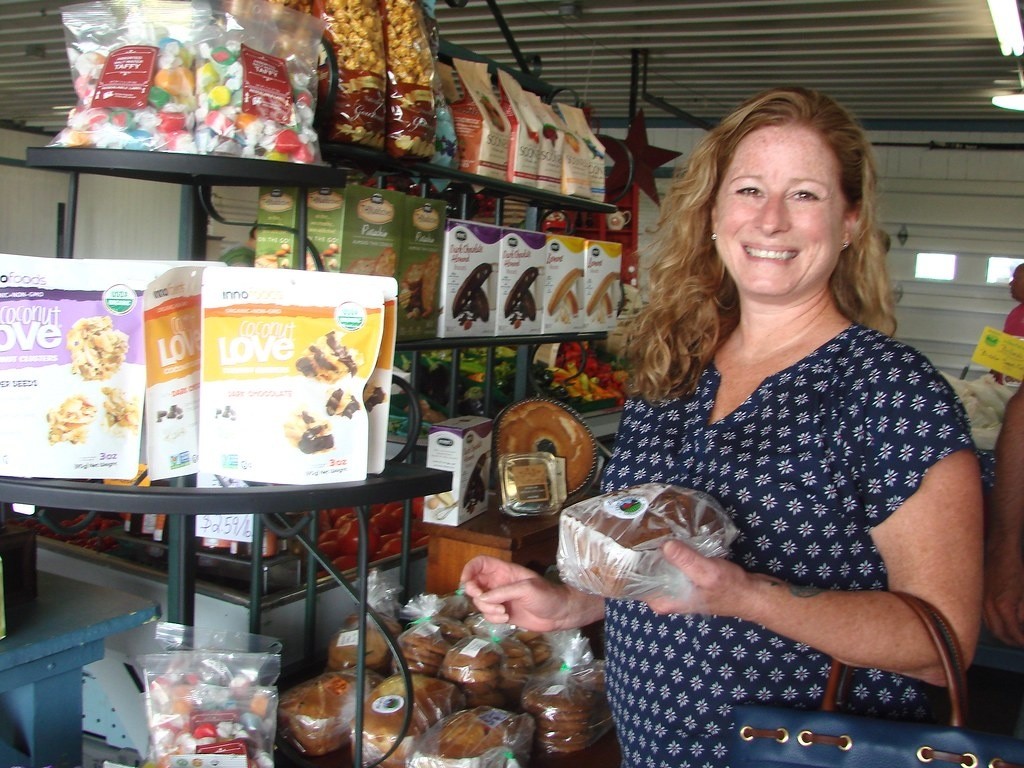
[289,497,431,583]
[6,513,126,555]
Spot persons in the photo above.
[983,264,1024,648]
[459,82,984,768]
[220,222,259,268]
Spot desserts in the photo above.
[48,315,142,444]
[283,331,390,454]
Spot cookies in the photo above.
[327,593,612,754]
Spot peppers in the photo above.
[388,341,636,438]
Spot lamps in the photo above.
[987,0,1024,111]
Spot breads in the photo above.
[254,242,619,323]
[461,452,487,508]
[564,487,726,597]
[495,400,594,492]
[278,665,535,768]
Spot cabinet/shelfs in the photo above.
[341,35,623,450]
[0,144,455,652]
[423,503,561,595]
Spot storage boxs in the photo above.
[497,224,547,338]
[398,194,447,343]
[306,184,404,282]
[422,415,493,527]
[252,184,304,268]
[583,239,625,332]
[542,231,585,335]
[437,218,503,339]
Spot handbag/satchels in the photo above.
[733,592,1024,768]
